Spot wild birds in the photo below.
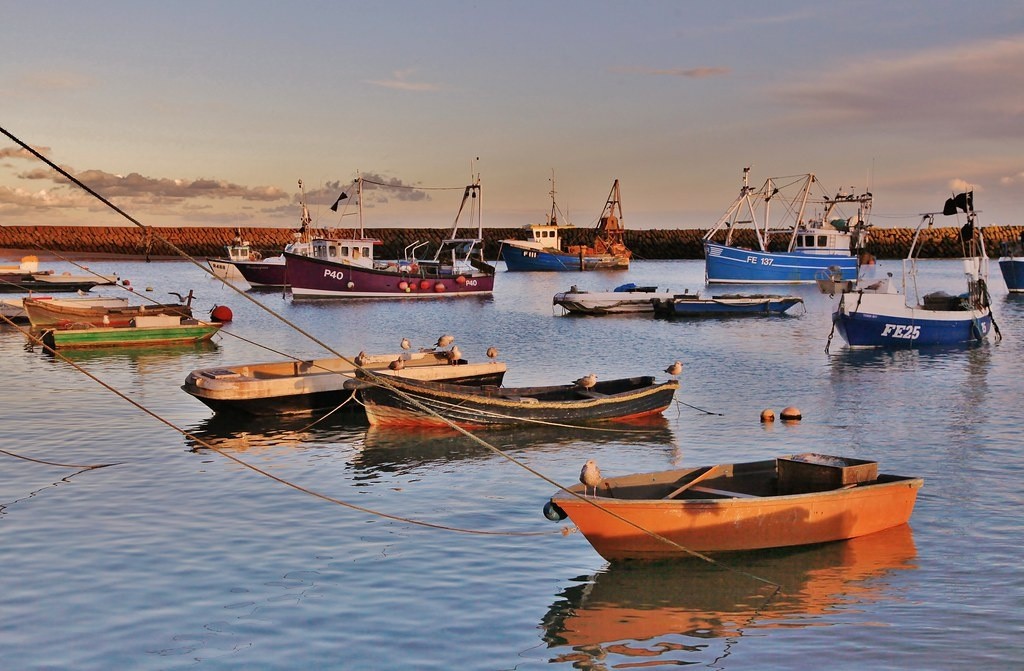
[571,373,598,392]
[486,347,498,364]
[102,315,110,327]
[354,351,367,367]
[400,338,412,354]
[579,458,602,499]
[77,289,90,298]
[445,346,461,366]
[662,361,683,380]
[387,356,404,376]
[432,335,455,351]
[138,304,145,313]
[418,347,436,353]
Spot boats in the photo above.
[830,186,990,349]
[550,451,925,565]
[997,231,1024,292]
[552,282,701,312]
[0,255,224,351]
[344,350,680,431]
[283,157,496,305]
[498,168,630,272]
[225,180,328,291]
[702,166,871,287]
[652,289,801,319]
[206,209,264,279]
[181,352,506,417]
